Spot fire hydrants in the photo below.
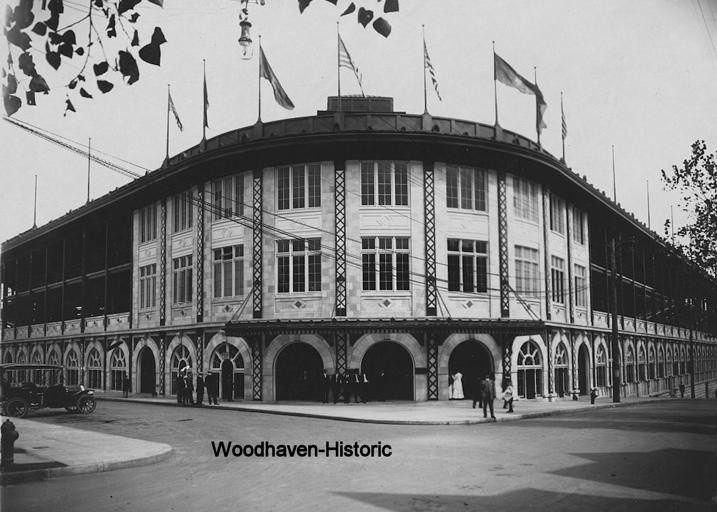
[1,419,19,463]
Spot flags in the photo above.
[424,42,442,103]
[338,37,363,85]
[536,86,547,134]
[203,79,210,129]
[261,47,295,110]
[169,95,184,132]
[494,52,546,107]
[561,109,569,140]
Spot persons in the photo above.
[501,376,511,409]
[172,371,220,406]
[122,374,130,398]
[450,369,465,400]
[503,382,514,412]
[320,369,377,404]
[480,373,497,419]
[472,374,483,409]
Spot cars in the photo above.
[1,363,97,418]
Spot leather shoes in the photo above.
[473,404,513,418]
